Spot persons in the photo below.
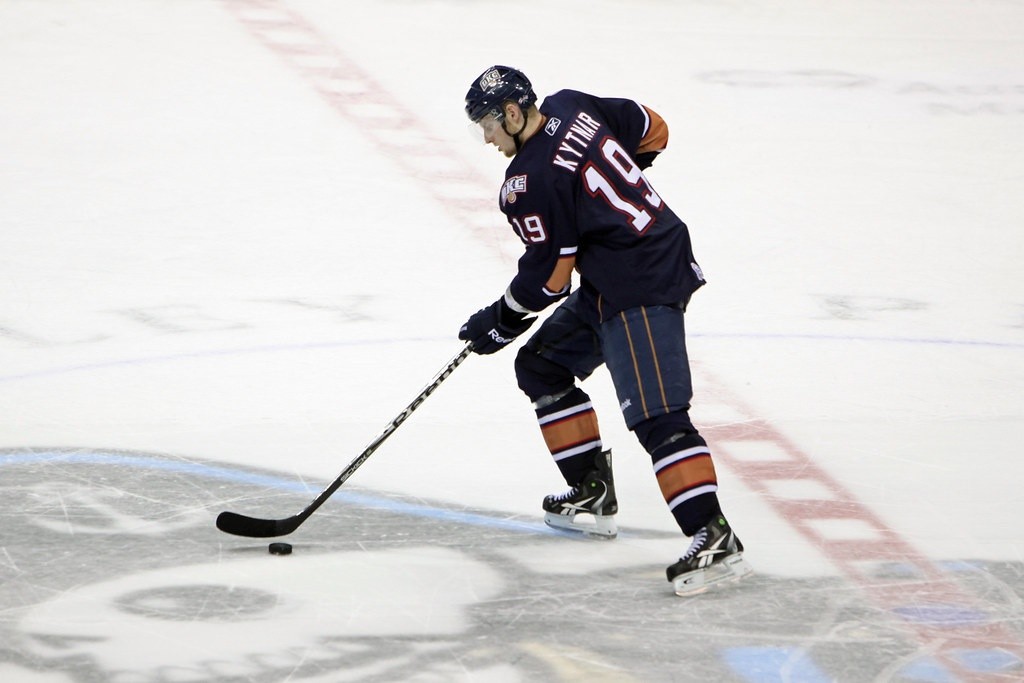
[459,65,755,597]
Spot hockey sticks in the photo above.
[215,340,476,539]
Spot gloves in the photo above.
[458,294,538,355]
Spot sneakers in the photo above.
[666,515,754,597]
[542,447,619,541]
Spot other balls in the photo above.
[268,543,293,555]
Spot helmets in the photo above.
[465,64,537,124]
